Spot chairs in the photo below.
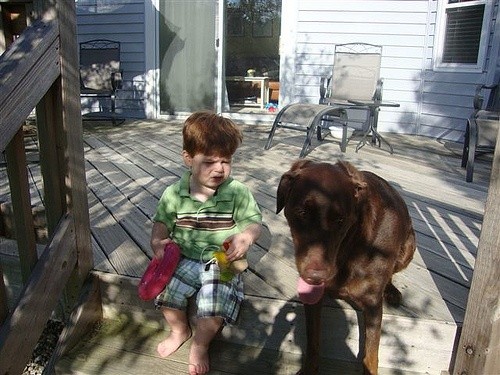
[460,72,500,112]
[80,40,126,126]
[317,43,385,145]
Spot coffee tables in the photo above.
[225,75,270,112]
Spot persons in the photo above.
[151,112,263,375]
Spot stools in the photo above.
[461,116,500,182]
[264,101,347,158]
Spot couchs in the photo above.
[227,55,281,99]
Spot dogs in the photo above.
[276,159,416,375]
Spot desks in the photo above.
[347,99,400,154]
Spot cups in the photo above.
[247,69,256,77]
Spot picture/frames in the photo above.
[227,7,246,38]
[252,10,273,38]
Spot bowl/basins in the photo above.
[329,126,355,138]
